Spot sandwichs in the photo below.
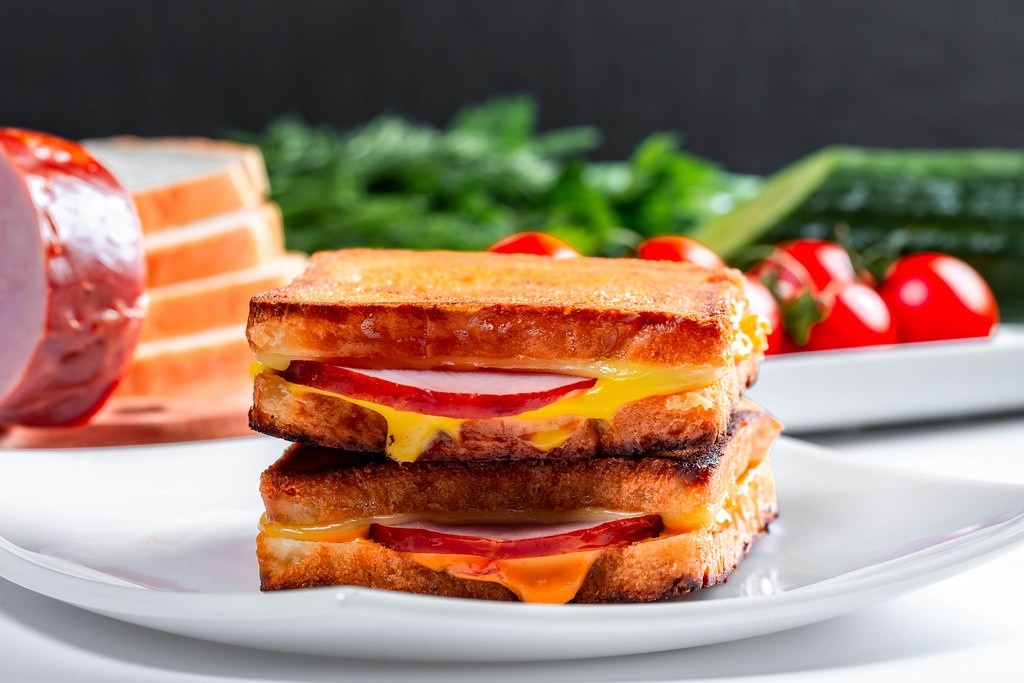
[246,248,787,605]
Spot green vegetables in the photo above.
[258,93,750,251]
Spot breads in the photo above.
[68,134,313,399]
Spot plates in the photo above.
[739,314,1024,440]
[1,434,1022,662]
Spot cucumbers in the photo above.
[683,146,1024,323]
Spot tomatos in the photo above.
[484,230,999,357]
